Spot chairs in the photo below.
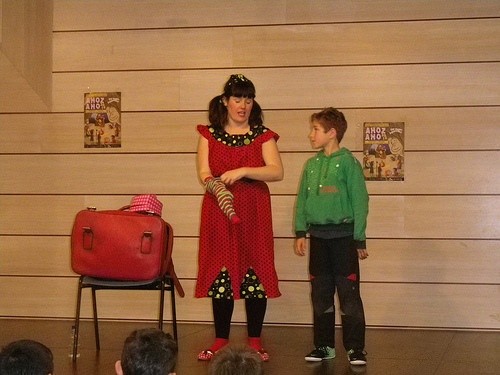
[73,275,178,363]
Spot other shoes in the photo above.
[258,350,270,361]
[197,340,231,361]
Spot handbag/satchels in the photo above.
[71,205,185,298]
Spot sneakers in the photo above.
[346,348,368,365]
[304,346,336,362]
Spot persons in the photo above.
[197,74,283,362]
[294,108,369,364]
[0,328,265,374]
